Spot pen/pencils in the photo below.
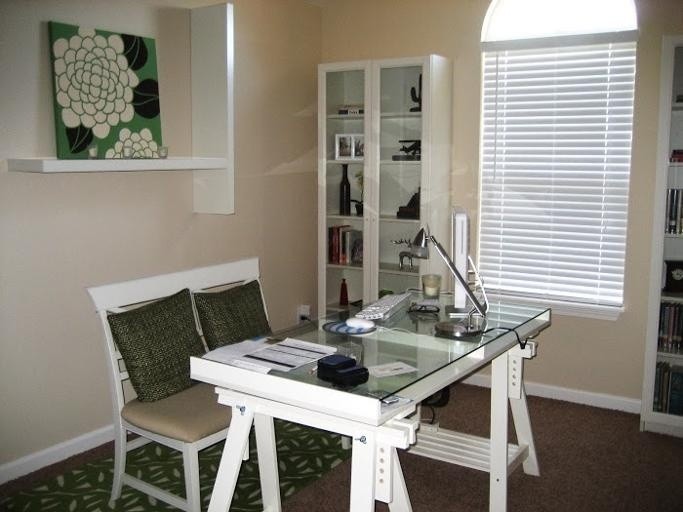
[309,366,318,374]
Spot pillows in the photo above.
[194,281,273,351]
[106,288,207,403]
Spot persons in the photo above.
[338,137,363,155]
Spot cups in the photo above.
[421,273,440,298]
[337,341,361,366]
[158,146,168,158]
[87,144,97,159]
[122,146,132,159]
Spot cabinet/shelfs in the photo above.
[316,56,451,318]
[638,34,683,438]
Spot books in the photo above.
[328,224,363,265]
[653,93,683,416]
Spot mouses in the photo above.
[346,316,375,329]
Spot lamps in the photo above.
[410,229,485,335]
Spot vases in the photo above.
[340,278,348,307]
[355,204,363,217]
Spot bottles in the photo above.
[338,278,347,304]
[339,162,350,216]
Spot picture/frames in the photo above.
[335,133,364,160]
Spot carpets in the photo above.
[1,418,350,512]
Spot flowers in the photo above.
[350,170,363,203]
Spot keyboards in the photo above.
[355,293,410,319]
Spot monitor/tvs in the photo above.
[445,206,489,317]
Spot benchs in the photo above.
[88,259,350,512]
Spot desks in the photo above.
[189,294,552,512]
